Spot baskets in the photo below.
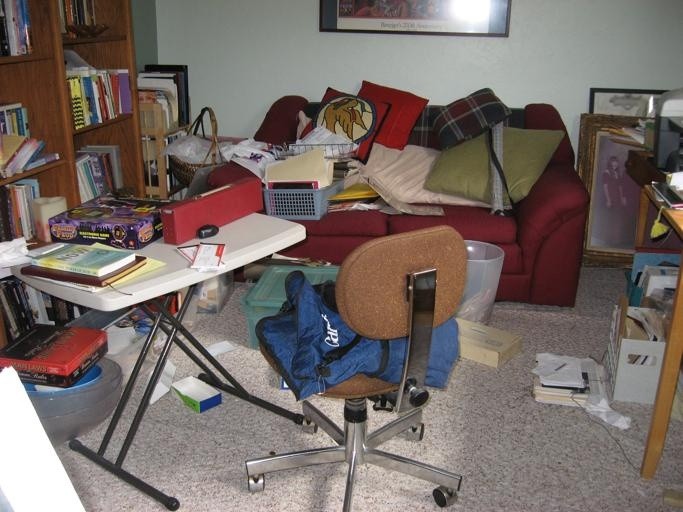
[273,144,361,158]
[263,180,345,221]
[169,107,224,186]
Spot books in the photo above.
[651,183,683,207]
[66,67,133,130]
[1,180,40,241]
[1,1,33,56]
[75,145,122,203]
[2,276,109,387]
[20,243,147,287]
[534,315,671,409]
[1,104,61,178]
[59,2,97,33]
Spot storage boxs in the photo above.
[245,263,340,349]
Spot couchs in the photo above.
[208,94,590,308]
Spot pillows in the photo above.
[297,80,566,212]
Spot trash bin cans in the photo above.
[453,239,505,326]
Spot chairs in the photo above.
[244,224,468,512]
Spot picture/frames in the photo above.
[576,86,670,268]
[318,0,512,38]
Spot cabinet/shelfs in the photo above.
[0,0,145,355]
[139,93,192,202]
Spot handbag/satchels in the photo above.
[254,269,459,402]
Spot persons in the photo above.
[601,157,639,247]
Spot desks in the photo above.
[627,182,682,479]
[9,212,308,511]
